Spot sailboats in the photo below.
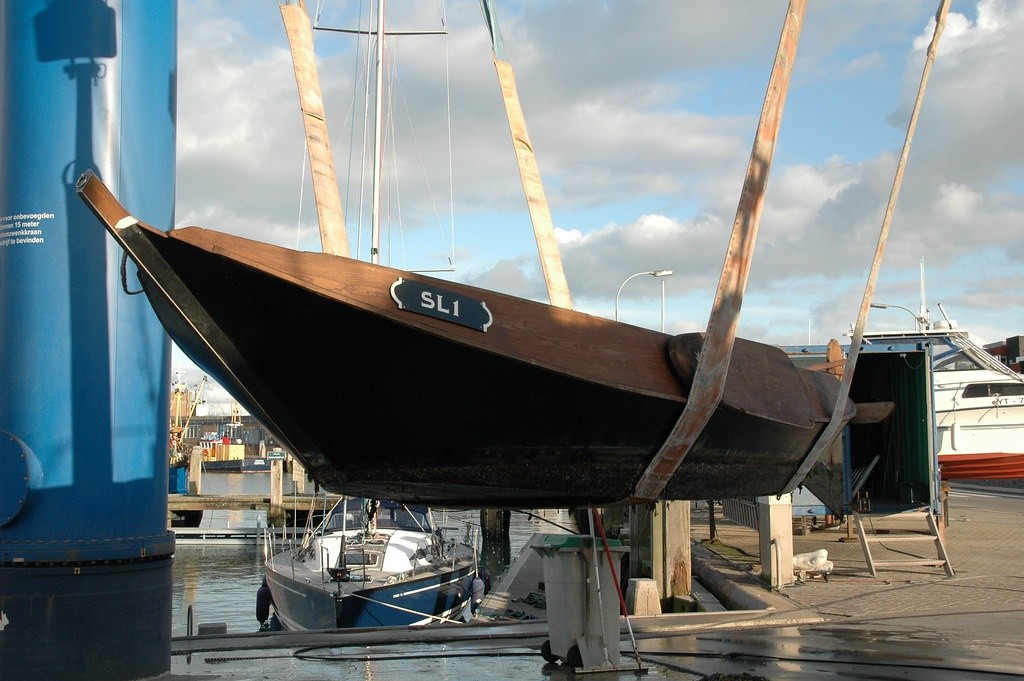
[252,1,489,633]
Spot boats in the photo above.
[844,252,1024,486]
[191,398,270,473]
[167,370,209,495]
[74,1,863,509]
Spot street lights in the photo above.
[614,270,674,324]
